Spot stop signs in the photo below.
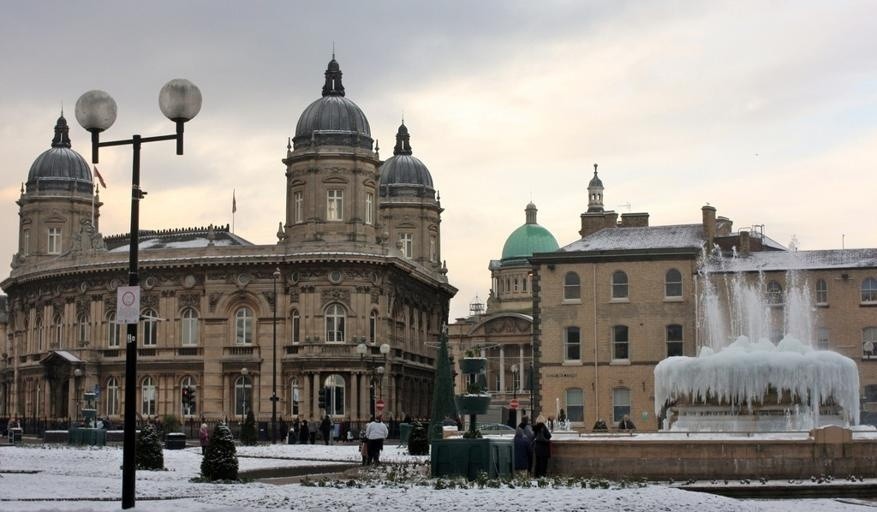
[510,400,518,408]
[375,400,383,410]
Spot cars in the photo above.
[455,423,514,435]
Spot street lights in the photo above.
[510,364,518,399]
[75,80,203,507]
[239,367,248,428]
[356,343,390,422]
[271,270,280,423]
[377,366,384,416]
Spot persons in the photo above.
[512,414,533,476]
[358,417,372,466]
[197,421,208,455]
[617,414,635,433]
[365,412,389,463]
[532,414,552,478]
[280,409,335,446]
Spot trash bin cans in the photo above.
[259,422,268,441]
